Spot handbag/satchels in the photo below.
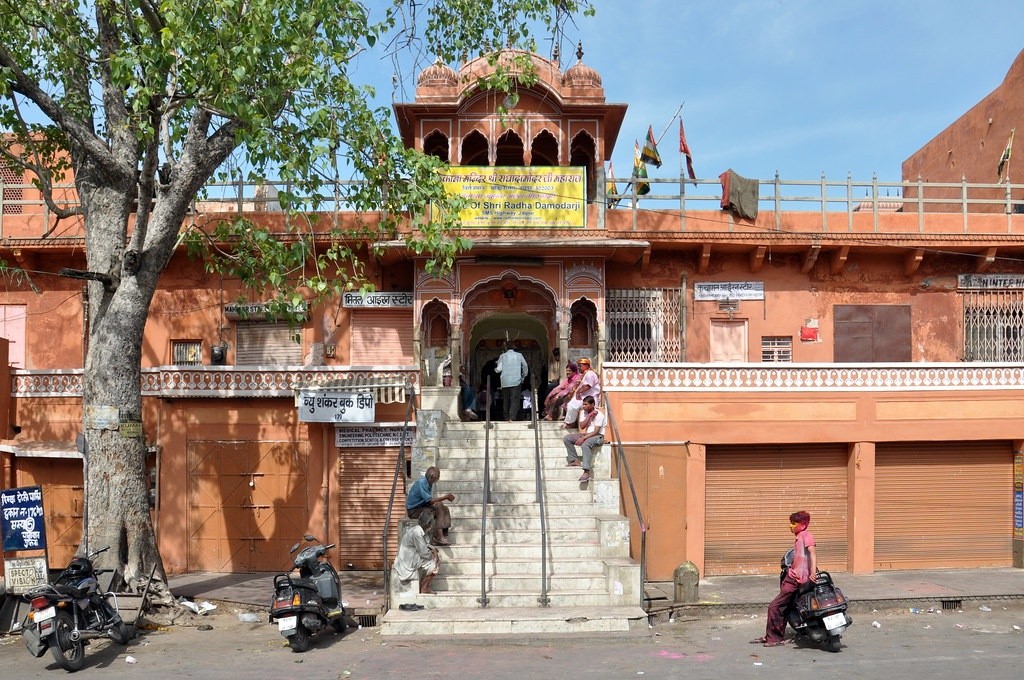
[20,612,49,657]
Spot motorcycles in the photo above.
[20,546,131,672]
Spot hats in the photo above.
[577,357,590,364]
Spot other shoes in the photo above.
[748,637,767,643]
[544,415,553,421]
[578,473,591,482]
[399,603,425,611]
[763,641,784,647]
[566,462,582,466]
[433,537,450,545]
[463,409,478,419]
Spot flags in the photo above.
[606,160,620,209]
[680,118,698,187]
[632,125,663,202]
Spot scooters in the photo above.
[779,549,853,653]
[271,534,347,653]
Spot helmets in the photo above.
[67,557,92,578]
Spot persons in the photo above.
[495,342,529,421]
[750,510,817,648]
[538,347,583,421]
[564,396,605,481]
[392,509,442,594]
[459,365,479,421]
[561,357,600,429]
[404,466,455,547]
